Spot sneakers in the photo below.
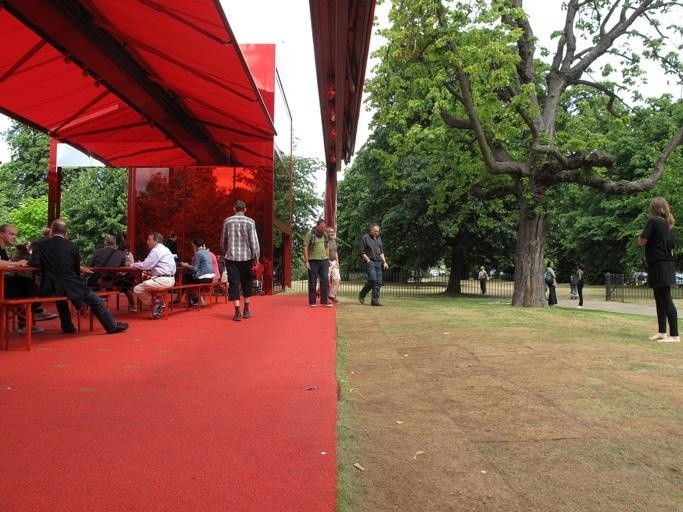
[372,301,383,306]
[244,310,250,318]
[33,309,58,321]
[359,293,365,304]
[192,301,206,306]
[18,325,44,333]
[169,299,180,306]
[329,297,337,302]
[130,305,137,312]
[233,312,241,321]
[151,303,166,321]
[113,323,129,333]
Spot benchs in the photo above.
[0,283,228,351]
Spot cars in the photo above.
[428,268,446,277]
[674,272,683,288]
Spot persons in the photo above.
[221,200,260,321]
[638,197,680,343]
[478,266,489,294]
[0,219,221,334]
[543,262,585,309]
[303,220,333,306]
[317,228,341,302]
[359,223,388,306]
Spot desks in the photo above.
[0,266,184,334]
